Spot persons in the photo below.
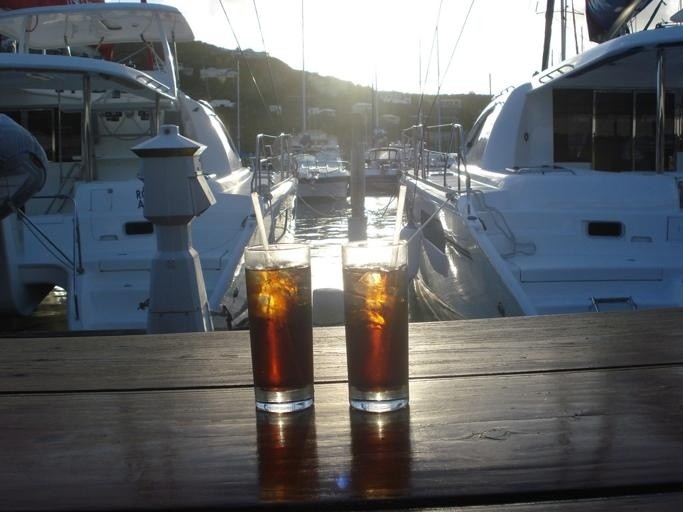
[0,38,17,52]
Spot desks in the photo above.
[1,306,682,512]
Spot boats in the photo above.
[0,0,300,335]
[398,1,683,321]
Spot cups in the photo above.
[338,241,413,413]
[240,241,315,413]
[248,408,325,501]
[347,407,411,496]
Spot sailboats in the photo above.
[247,1,457,200]
